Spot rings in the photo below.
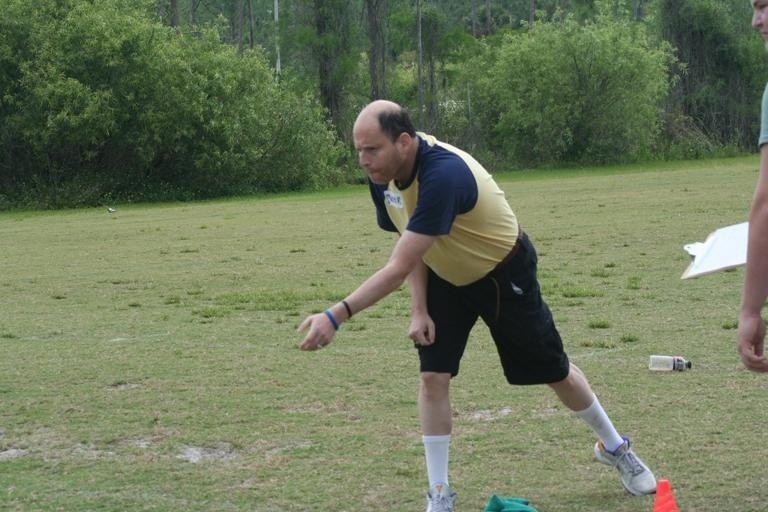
[317,344,322,349]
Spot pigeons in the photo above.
[107,206,116,213]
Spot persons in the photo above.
[298,98,658,511]
[736,1,768,373]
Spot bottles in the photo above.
[649,355,693,372]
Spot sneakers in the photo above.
[594,436,657,495]
[426,485,458,510]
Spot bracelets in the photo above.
[324,310,340,330]
[343,300,352,318]
